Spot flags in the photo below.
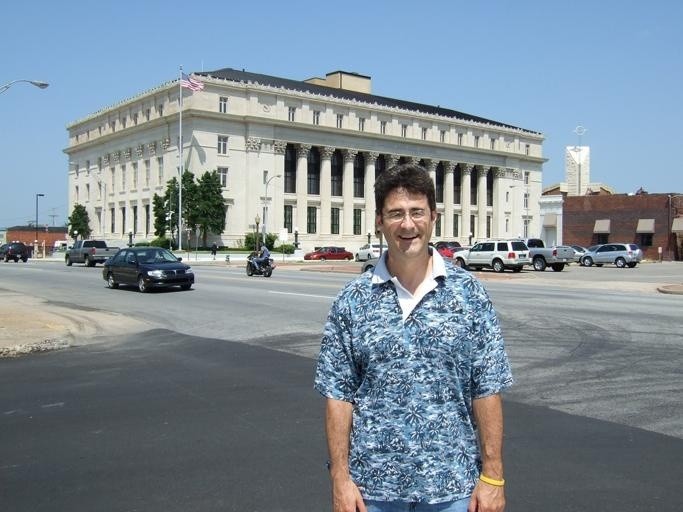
[181,72,204,92]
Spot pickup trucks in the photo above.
[506,238,574,272]
[64,239,120,267]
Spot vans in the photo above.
[52,241,67,252]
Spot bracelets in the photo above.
[479,474,505,486]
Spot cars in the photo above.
[548,245,591,266]
[303,247,354,262]
[431,241,470,263]
[354,243,388,262]
[587,244,604,253]
[360,256,379,274]
[102,245,194,293]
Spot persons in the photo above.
[314,163,515,512]
[252,242,270,271]
[210,242,218,260]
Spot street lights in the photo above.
[72,230,78,243]
[364,229,374,244]
[468,232,472,246]
[264,174,282,233]
[126,228,134,247]
[35,193,44,240]
[518,232,521,239]
[0,79,49,96]
[292,226,302,251]
[254,212,261,253]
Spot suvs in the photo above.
[580,243,644,268]
[0,242,28,263]
[451,237,533,273]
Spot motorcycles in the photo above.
[245,241,276,278]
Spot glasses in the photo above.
[388,210,426,222]
